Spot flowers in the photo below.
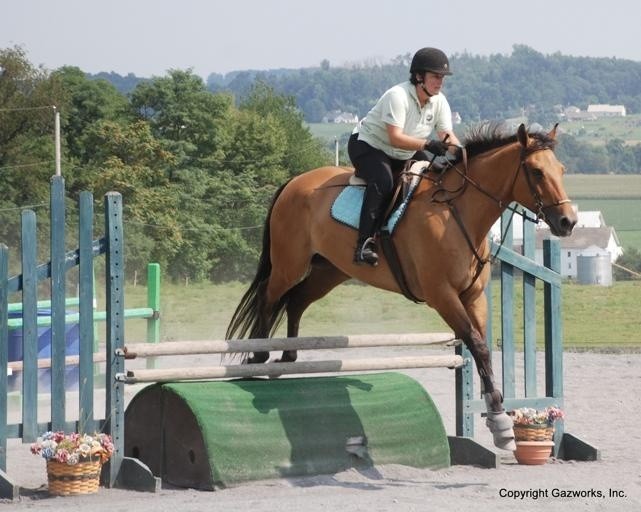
[30,431,115,464]
[511,406,565,425]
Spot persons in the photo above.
[348,46,463,268]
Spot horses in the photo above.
[217,120,579,453]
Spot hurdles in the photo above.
[0,175,601,499]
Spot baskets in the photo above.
[513,423,553,443]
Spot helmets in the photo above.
[410,47,455,76]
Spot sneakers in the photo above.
[354,237,379,264]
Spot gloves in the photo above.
[424,140,448,157]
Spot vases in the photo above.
[515,441,556,465]
[513,425,556,441]
[47,462,103,494]
